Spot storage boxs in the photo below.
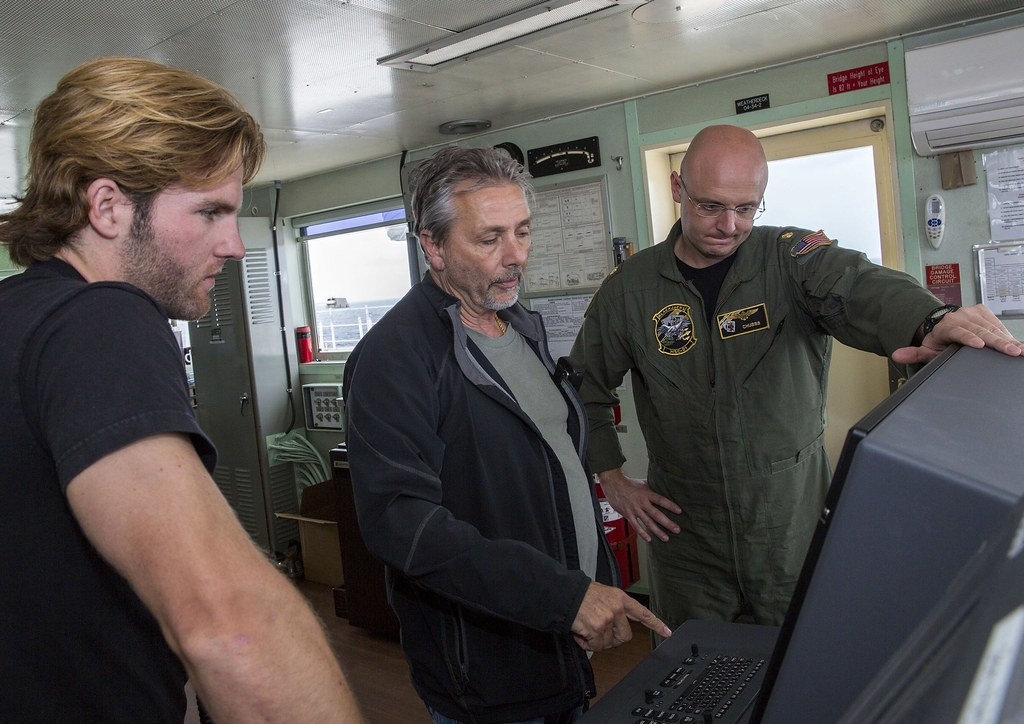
[276,511,345,586]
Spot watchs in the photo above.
[924,304,961,336]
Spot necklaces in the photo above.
[494,314,504,334]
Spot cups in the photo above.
[297,328,315,362]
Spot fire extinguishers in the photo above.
[592,473,631,593]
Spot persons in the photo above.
[566,125,1024,647]
[339,148,672,723]
[0,58,367,724]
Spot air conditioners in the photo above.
[905,23,1024,157]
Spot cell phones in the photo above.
[556,357,584,391]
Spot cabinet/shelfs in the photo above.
[190,218,311,577]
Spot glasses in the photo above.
[679,174,766,222]
